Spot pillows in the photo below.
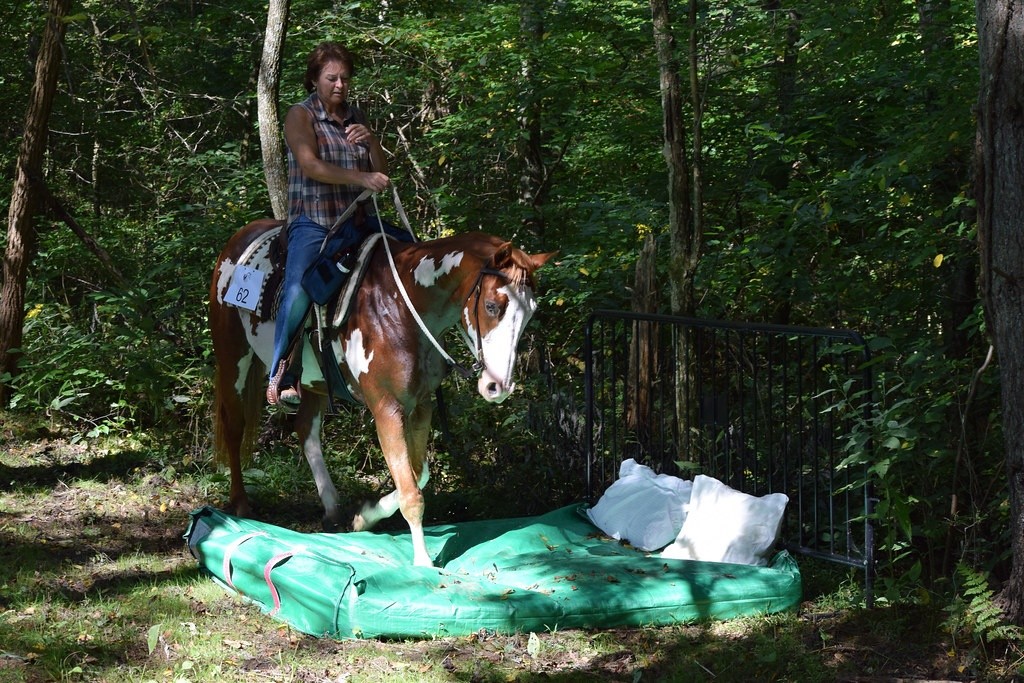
[644,474,789,566]
[577,457,696,554]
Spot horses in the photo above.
[208,219,561,568]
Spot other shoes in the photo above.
[281,386,300,405]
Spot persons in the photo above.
[266,42,420,417]
